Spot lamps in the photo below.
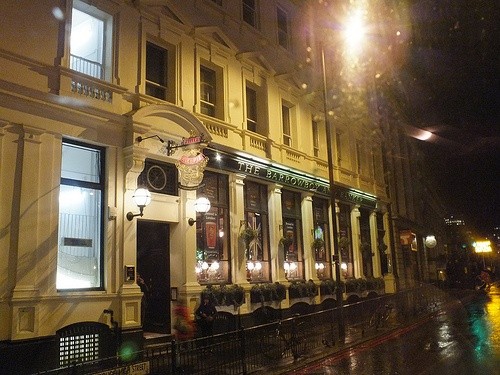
[188,197,211,226]
[127,188,152,221]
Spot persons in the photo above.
[195,295,218,343]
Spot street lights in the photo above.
[320,4,384,336]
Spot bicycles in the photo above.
[259,313,315,359]
[369,300,400,330]
